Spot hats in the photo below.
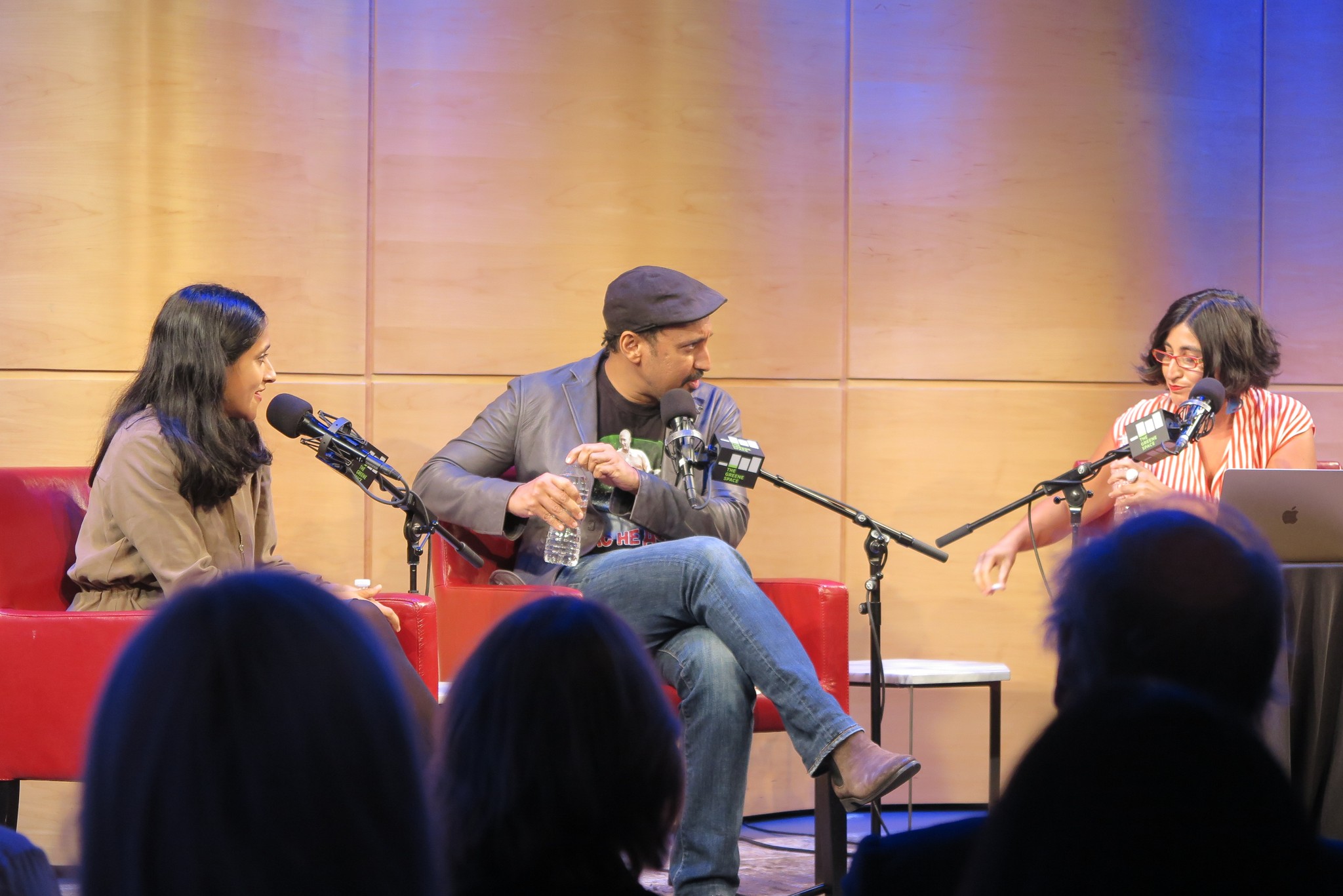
[603,265,728,336]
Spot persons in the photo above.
[67,284,439,768]
[846,511,1343,896]
[414,265,921,896]
[972,288,1319,593]
[80,573,687,896]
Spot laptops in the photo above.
[1216,469,1343,562]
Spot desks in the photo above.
[848,659,1009,810]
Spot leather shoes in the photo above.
[825,730,921,813]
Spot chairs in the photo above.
[0,467,437,831]
[430,465,850,896]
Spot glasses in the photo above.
[1152,347,1205,370]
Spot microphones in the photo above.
[661,388,697,509]
[1173,378,1226,455]
[266,394,401,481]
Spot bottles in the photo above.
[544,458,589,567]
[354,579,370,591]
[1110,458,1141,521]
